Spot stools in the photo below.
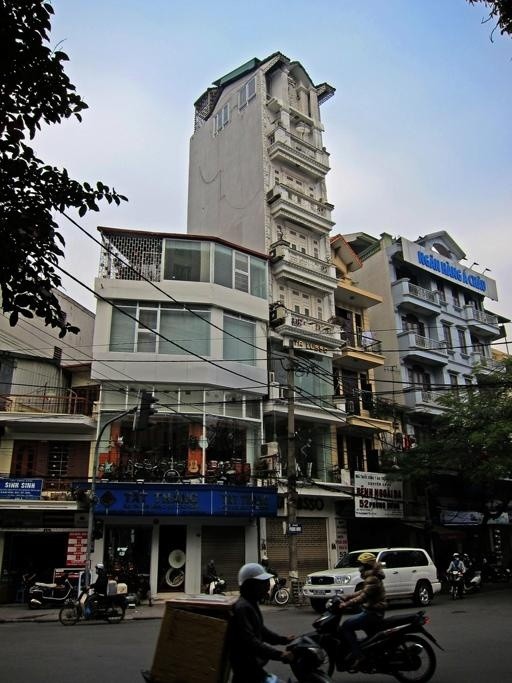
[16,589,27,602]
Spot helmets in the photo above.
[453,553,459,556]
[96,564,104,570]
[357,552,376,564]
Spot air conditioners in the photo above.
[271,308,285,322]
[266,187,281,204]
[259,442,278,459]
[269,246,283,261]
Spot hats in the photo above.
[238,563,274,586]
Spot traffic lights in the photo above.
[142,390,159,432]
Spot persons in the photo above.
[271,434,314,478]
[261,557,269,572]
[333,551,386,667]
[205,559,217,581]
[408,438,421,449]
[444,551,512,601]
[84,563,109,616]
[225,562,296,683]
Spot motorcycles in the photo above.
[140,633,333,683]
[27,573,74,610]
[259,569,289,605]
[308,589,446,682]
[446,567,465,600]
[463,570,482,594]
[202,573,225,595]
[58,581,129,625]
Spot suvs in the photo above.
[301,546,442,610]
